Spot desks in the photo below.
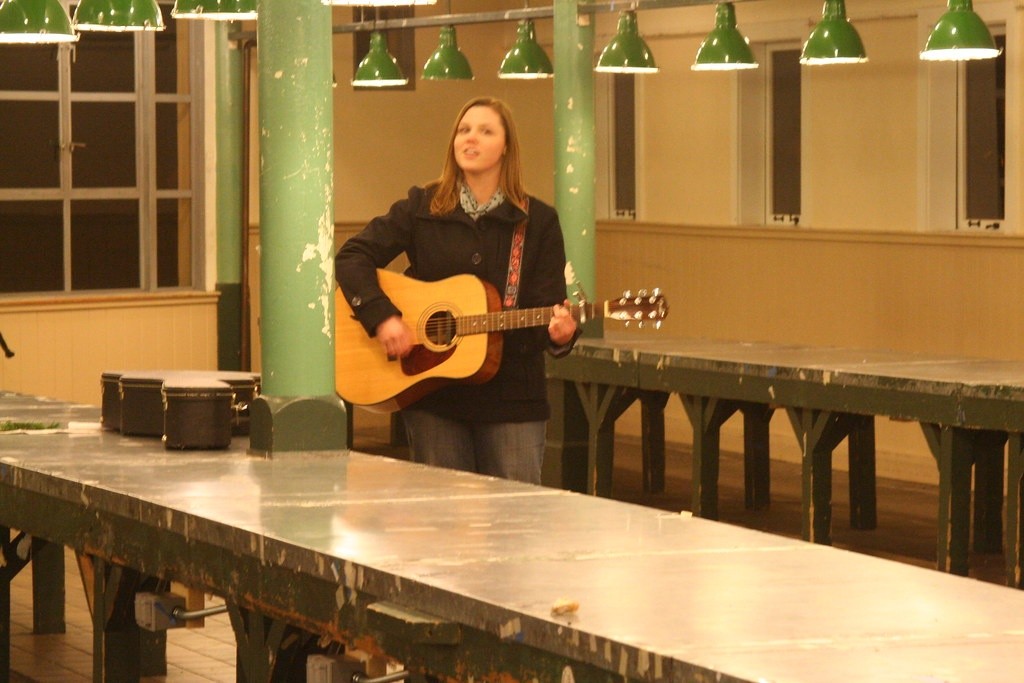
[542,337,1024,587]
[0,389,1024,683]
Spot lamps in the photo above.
[497,20,554,79]
[799,0,868,66]
[350,31,406,86]
[72,0,166,32]
[422,26,474,81]
[690,3,759,71]
[170,0,258,20]
[594,8,659,73]
[919,0,999,61]
[0,0,77,44]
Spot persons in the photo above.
[334,96,583,485]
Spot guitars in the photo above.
[334,268,671,418]
